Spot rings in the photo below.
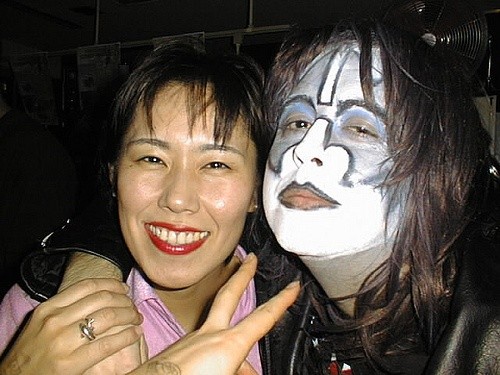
[78,314,95,341]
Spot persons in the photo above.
[18,11,500,375]
[1,36,264,375]
